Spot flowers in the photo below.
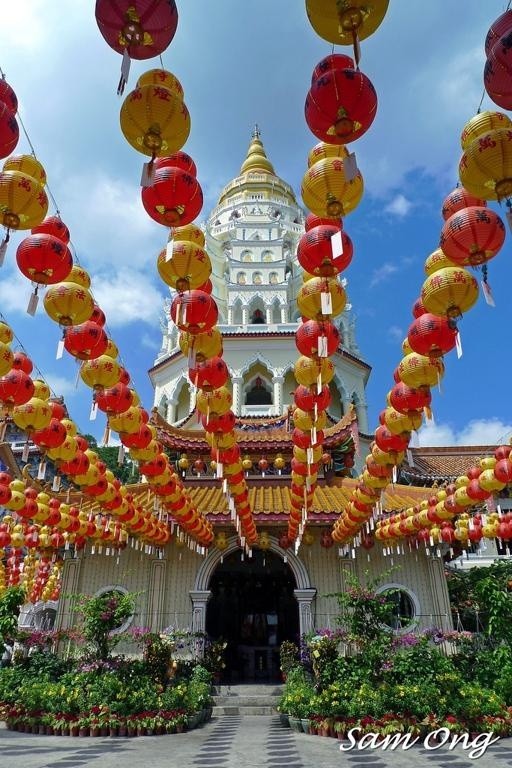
[315,625,474,650]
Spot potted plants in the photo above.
[271,634,512,745]
[0,651,213,737]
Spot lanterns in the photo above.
[0,550,61,604]
[0,0,512,550]
